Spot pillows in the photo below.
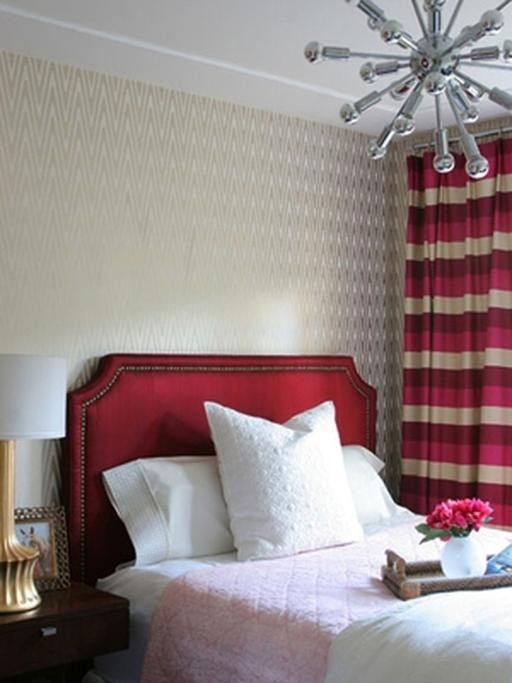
[204,401,364,561]
[102,444,412,567]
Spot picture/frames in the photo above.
[14,506,71,592]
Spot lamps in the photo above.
[303,0,511,178]
[1,354,66,613]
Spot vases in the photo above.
[440,536,487,578]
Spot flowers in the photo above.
[415,497,495,544]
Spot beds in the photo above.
[65,353,512,682]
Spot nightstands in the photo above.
[1,582,130,683]
